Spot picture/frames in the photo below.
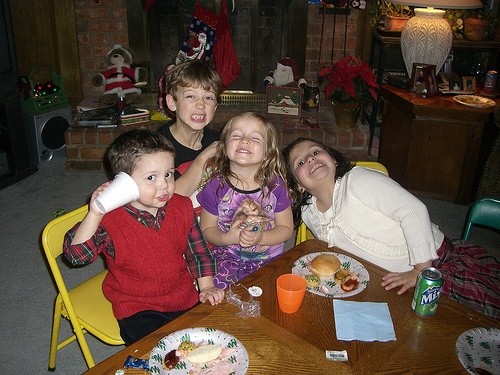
[449,73,462,90]
[408,62,439,98]
[268,86,306,116]
[463,75,476,91]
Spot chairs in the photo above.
[42,204,126,373]
[294,161,388,245]
[463,195,500,248]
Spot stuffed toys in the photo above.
[91,47,148,103]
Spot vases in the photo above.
[335,97,363,128]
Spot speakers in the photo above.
[22,105,73,166]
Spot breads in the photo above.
[310,254,341,277]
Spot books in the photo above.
[77,95,107,113]
[121,108,149,125]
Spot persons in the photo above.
[196,112,293,290]
[63,128,224,346]
[281,137,500,321]
[156,59,226,220]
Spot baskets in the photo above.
[19,63,67,114]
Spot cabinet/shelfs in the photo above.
[355,29,500,155]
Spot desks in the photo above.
[380,80,500,205]
[84,238,500,375]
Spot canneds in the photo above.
[483,70,498,95]
[412,267,444,316]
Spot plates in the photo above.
[291,252,369,299]
[149,327,249,375]
[453,95,495,108]
[455,326,500,375]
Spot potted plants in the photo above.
[380,3,500,43]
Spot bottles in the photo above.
[302,81,321,113]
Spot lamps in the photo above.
[379,0,484,79]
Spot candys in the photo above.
[123,355,149,370]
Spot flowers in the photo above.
[320,53,380,104]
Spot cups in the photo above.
[276,274,307,314]
[93,172,140,214]
